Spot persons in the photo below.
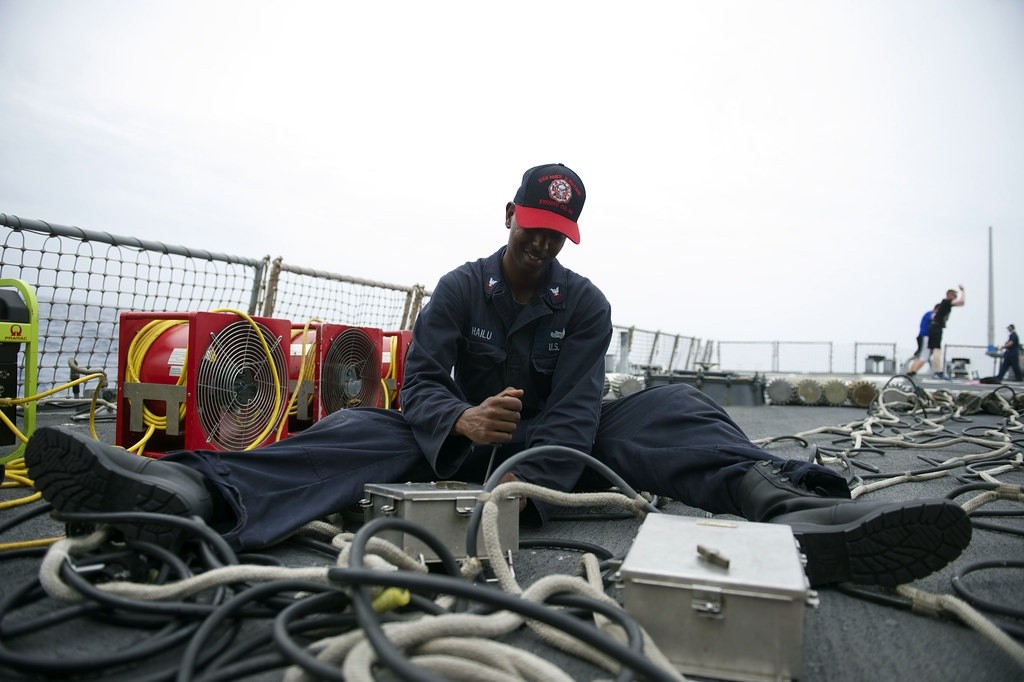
[907,284,967,381]
[911,303,941,377]
[995,324,1023,382]
[21,163,974,589]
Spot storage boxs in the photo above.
[359,480,520,565]
[609,511,809,681]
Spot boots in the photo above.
[732,456,973,589]
[25,427,216,560]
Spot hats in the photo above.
[513,163,586,244]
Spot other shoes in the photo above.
[933,371,951,380]
